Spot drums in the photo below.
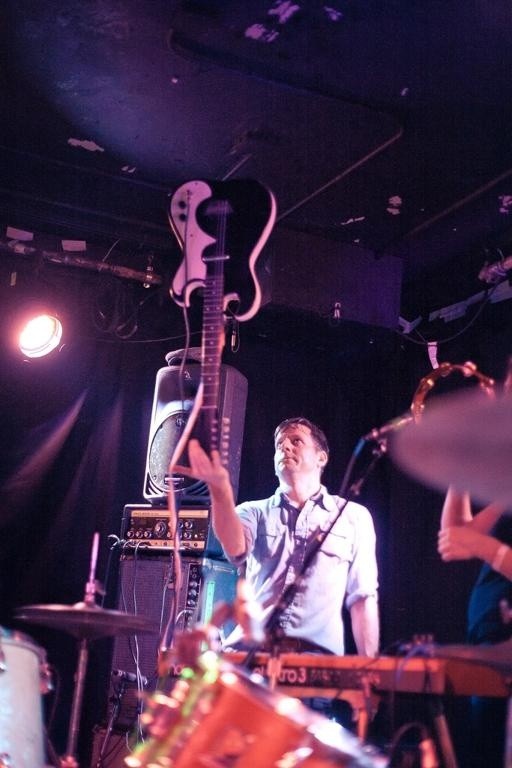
[0,626,55,768]
[124,648,392,767]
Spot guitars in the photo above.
[169,177,276,469]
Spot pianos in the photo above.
[218,649,510,698]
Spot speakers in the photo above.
[103,556,243,730]
[143,365,250,506]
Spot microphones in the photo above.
[231,319,240,353]
[368,407,423,442]
[114,669,147,686]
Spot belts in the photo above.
[261,635,337,655]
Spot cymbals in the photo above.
[13,603,155,638]
[392,385,512,515]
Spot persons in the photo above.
[170,417,381,724]
[437,483,512,768]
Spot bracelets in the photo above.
[492,542,509,572]
